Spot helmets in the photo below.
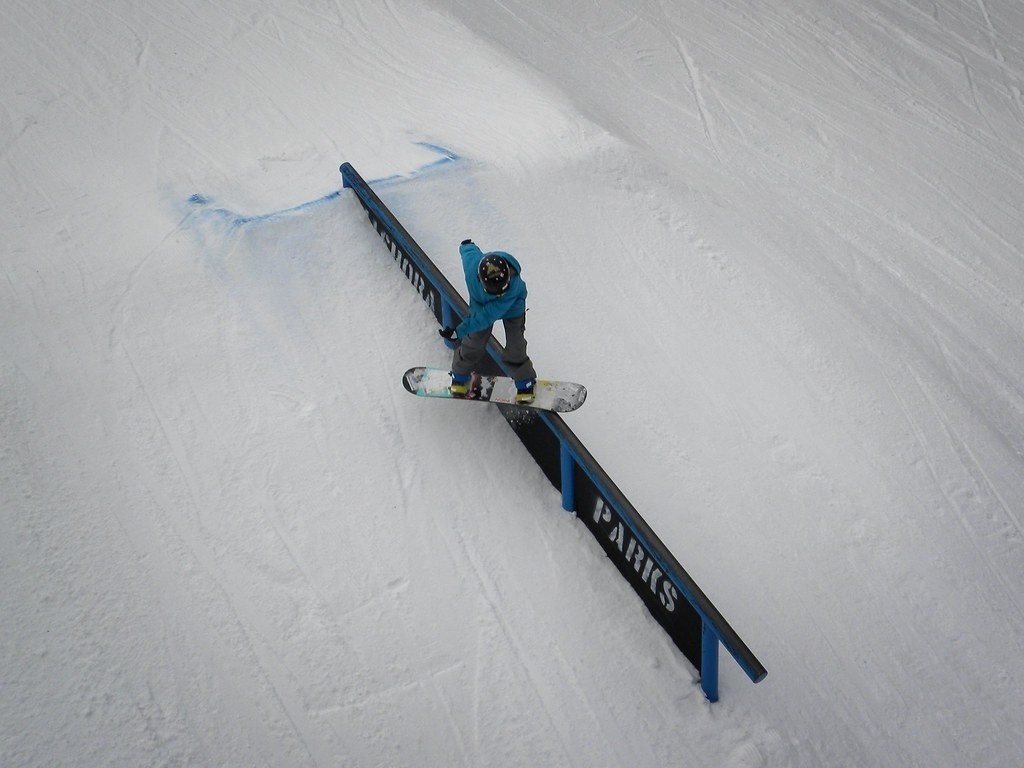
[478,255,510,295]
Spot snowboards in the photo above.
[402,367,589,413]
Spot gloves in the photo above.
[439,326,458,342]
[461,239,471,244]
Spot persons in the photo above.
[439,240,536,404]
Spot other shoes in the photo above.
[447,371,471,397]
[514,378,537,403]
